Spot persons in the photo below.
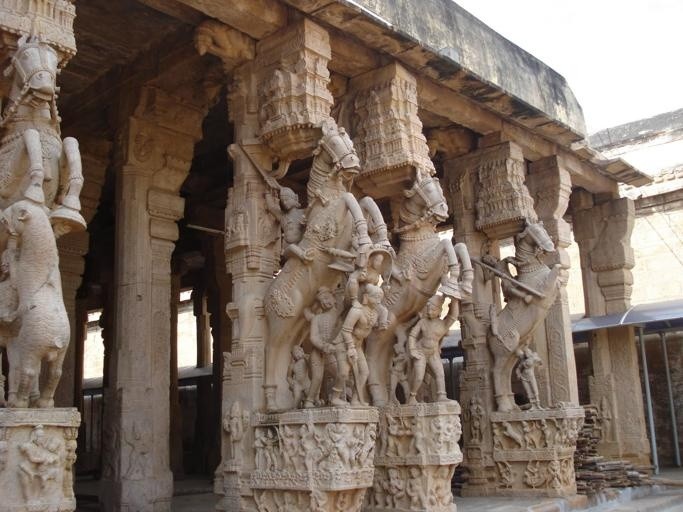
[251,422,376,512]
[597,397,611,443]
[463,398,578,490]
[481,254,534,304]
[513,347,546,411]
[291,249,459,407]
[0,425,77,504]
[265,178,314,263]
[368,413,463,511]
